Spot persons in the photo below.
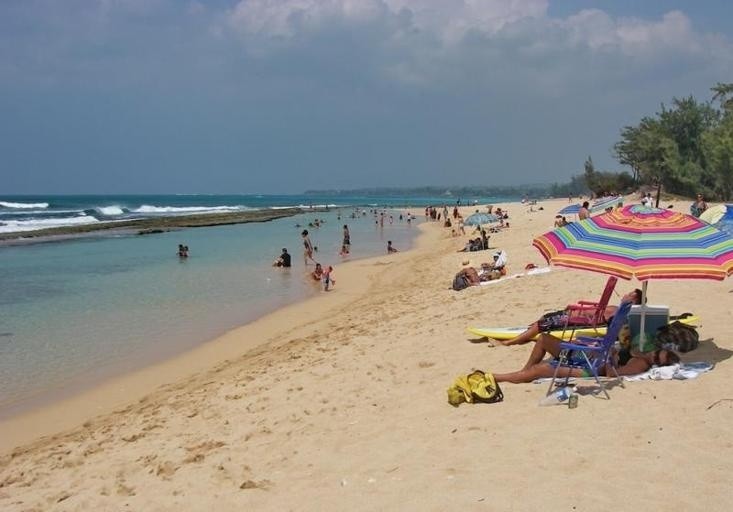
[272,197,510,291]
[494,288,648,346]
[492,332,680,384]
[642,192,646,205]
[690,192,709,218]
[181,245,190,258]
[617,193,624,208]
[178,244,184,256]
[641,192,653,208]
[577,201,590,220]
[568,191,573,203]
[553,214,572,228]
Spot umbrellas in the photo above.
[531,204,733,353]
[699,204,733,239]
[588,195,624,214]
[557,204,591,223]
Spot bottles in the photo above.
[538,385,576,407]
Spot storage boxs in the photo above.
[626,304,670,343]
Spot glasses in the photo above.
[653,349,661,365]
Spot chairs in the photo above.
[560,275,618,341]
[545,299,633,401]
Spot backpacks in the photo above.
[446,370,504,408]
